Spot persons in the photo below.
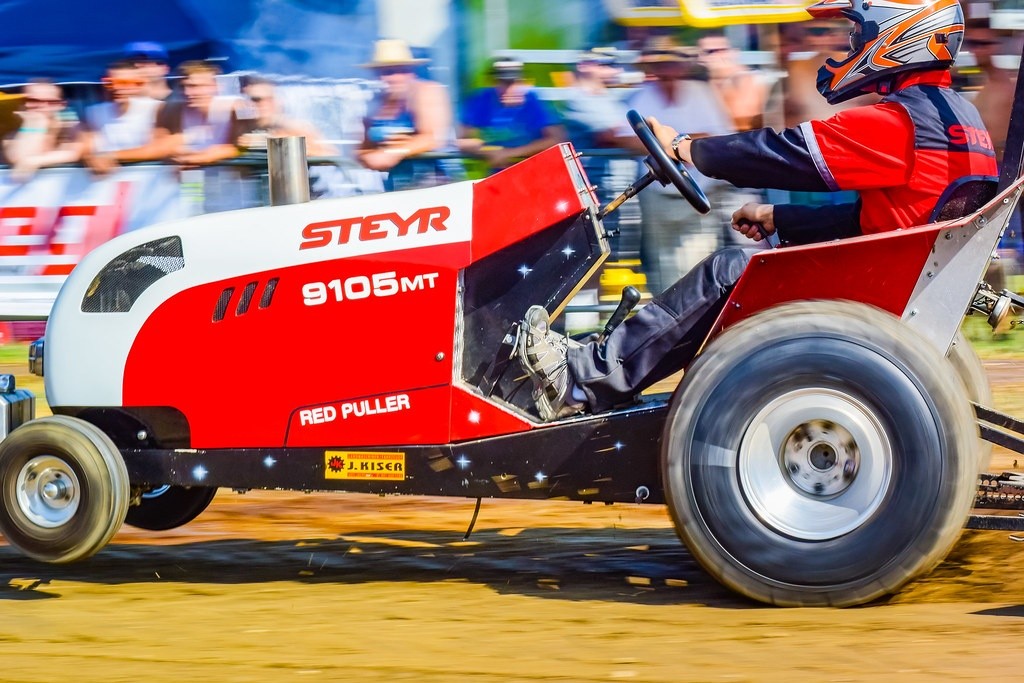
[0,42,328,168]
[519,1,999,424]
[457,56,564,173]
[354,40,465,192]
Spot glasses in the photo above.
[372,69,402,77]
[23,97,65,105]
[249,98,265,101]
[100,76,141,89]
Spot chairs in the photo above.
[930,176,1000,223]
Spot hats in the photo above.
[358,40,431,66]
[486,56,534,83]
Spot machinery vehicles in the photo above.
[0,46,1024,607]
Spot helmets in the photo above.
[804,1,962,104]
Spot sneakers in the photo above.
[515,306,586,425]
